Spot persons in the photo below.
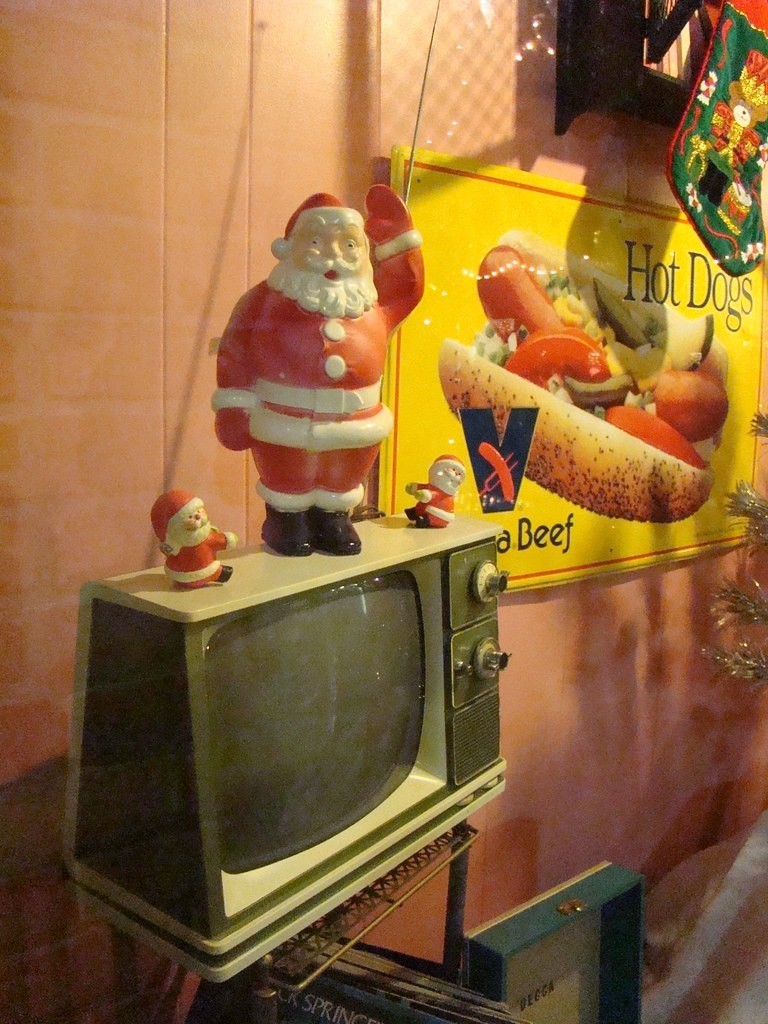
[211,182,424,556]
[404,454,466,528]
[149,489,240,586]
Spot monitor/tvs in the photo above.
[62,515,509,986]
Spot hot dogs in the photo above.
[439,231,730,522]
